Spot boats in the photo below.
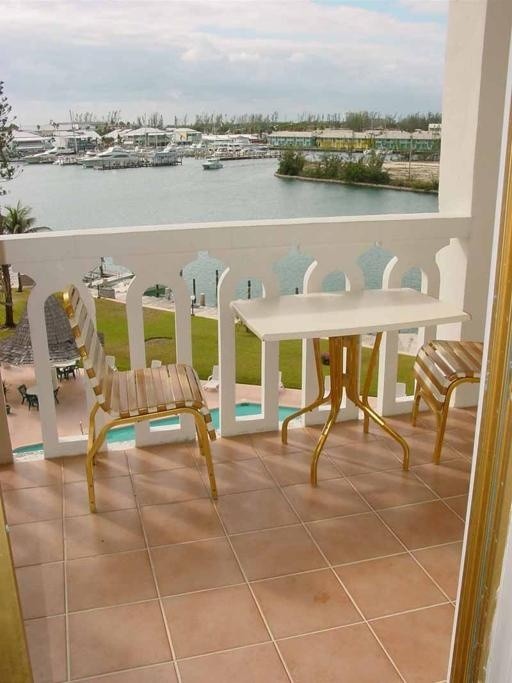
[5,138,268,170]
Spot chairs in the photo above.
[63,284,219,513]
[17,359,80,411]
[411,339,484,465]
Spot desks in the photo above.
[231,287,472,488]
